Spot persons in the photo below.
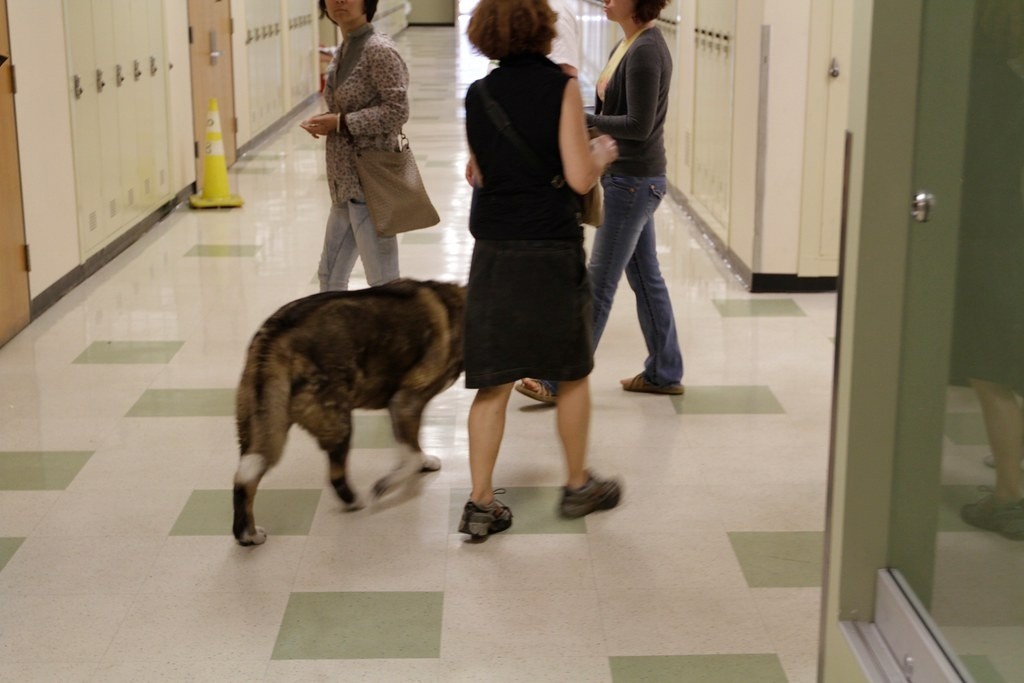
[954,0,1023,544]
[458,0,622,542]
[301,0,410,291]
[517,1,687,403]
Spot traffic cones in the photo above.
[190,97,245,209]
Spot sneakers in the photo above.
[560,476,617,518]
[458,487,513,539]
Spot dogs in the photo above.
[231,275,468,547]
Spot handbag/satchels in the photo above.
[581,172,605,228]
[353,140,440,236]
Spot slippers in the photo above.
[514,378,559,403]
[621,376,685,394]
